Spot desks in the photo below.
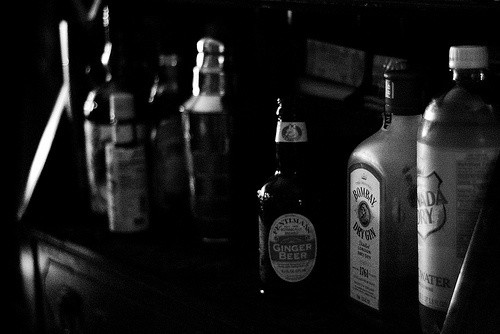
[19,218,246,332]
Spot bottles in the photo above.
[254,91,338,303]
[415,45,500,333]
[345,58,425,320]
[78,5,241,233]
